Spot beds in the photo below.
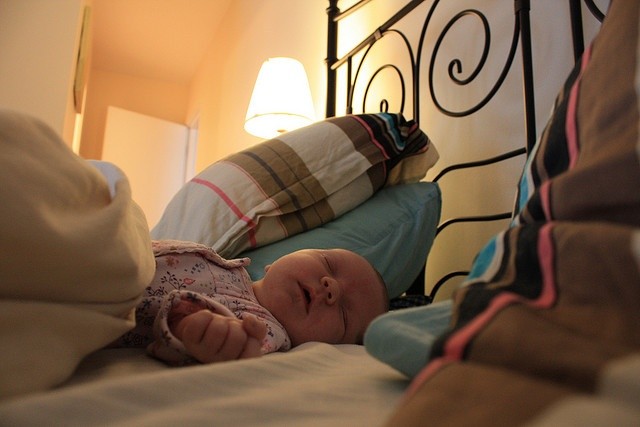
[1,1,634,424]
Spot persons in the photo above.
[104,241,389,368]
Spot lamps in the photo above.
[243,57,317,138]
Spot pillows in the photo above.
[149,114,439,261]
[242,182,442,300]
